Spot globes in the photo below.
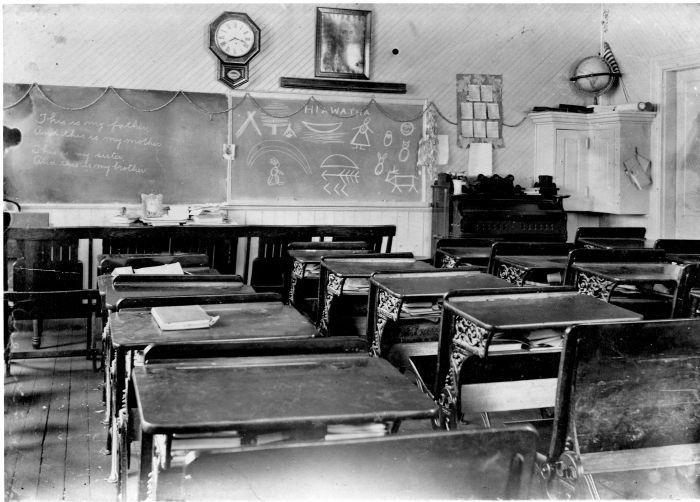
[569,55,616,109]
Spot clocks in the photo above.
[208,11,262,90]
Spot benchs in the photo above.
[3,225,396,349]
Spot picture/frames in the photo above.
[316,6,373,79]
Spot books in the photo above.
[113,205,224,229]
[151,304,213,330]
[399,301,442,320]
[305,265,320,276]
[168,420,387,453]
[488,330,564,352]
[116,262,185,277]
[614,284,640,295]
[342,278,371,295]
[526,281,550,287]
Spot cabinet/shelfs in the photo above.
[587,108,656,215]
[527,113,587,215]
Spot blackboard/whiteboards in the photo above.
[227,90,429,206]
[2,83,229,210]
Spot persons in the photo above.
[324,17,366,74]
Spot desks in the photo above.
[96,227,696,495]
